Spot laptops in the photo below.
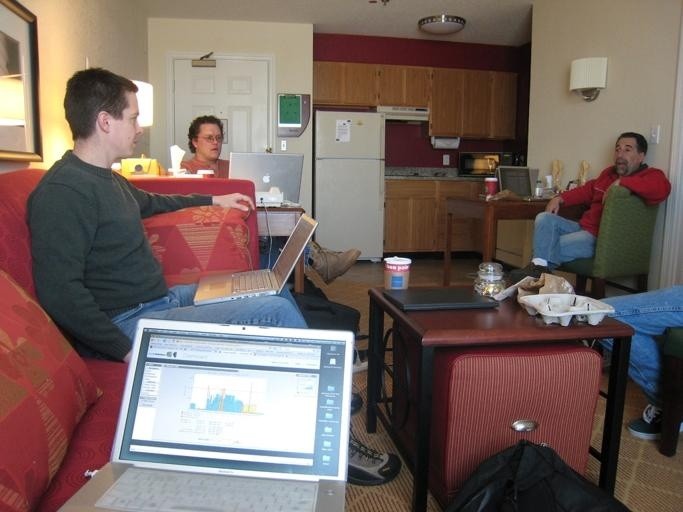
[498,166,553,202]
[228,151,305,204]
[58,317,355,512]
[192,213,319,306]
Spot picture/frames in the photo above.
[0,1,44,162]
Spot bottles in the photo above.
[474,262,506,298]
[534,180,544,197]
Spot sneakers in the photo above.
[350,391,364,416]
[625,401,683,441]
[346,422,403,486]
[507,261,554,286]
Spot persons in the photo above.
[173,113,360,285]
[520,132,670,279]
[27,66,401,484]
[588,285,683,439]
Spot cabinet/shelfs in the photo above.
[490,70,519,140]
[312,61,379,108]
[379,64,432,107]
[437,181,485,251]
[382,179,436,251]
[429,69,491,138]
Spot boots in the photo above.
[307,240,363,286]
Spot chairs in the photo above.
[556,185,660,298]
[656,324,682,457]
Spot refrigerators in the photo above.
[314,111,386,262]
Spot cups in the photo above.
[195,170,217,178]
[383,257,411,291]
[483,177,498,198]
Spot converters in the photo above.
[257,192,282,204]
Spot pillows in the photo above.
[144,202,256,286]
[0,268,102,510]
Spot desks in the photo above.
[255,205,308,293]
[441,197,590,286]
[365,285,634,512]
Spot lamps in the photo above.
[417,14,466,35]
[567,57,609,102]
[129,80,154,130]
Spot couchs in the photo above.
[0,166,261,512]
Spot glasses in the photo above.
[194,133,224,143]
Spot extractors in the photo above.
[373,106,428,125]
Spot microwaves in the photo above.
[458,151,516,177]
